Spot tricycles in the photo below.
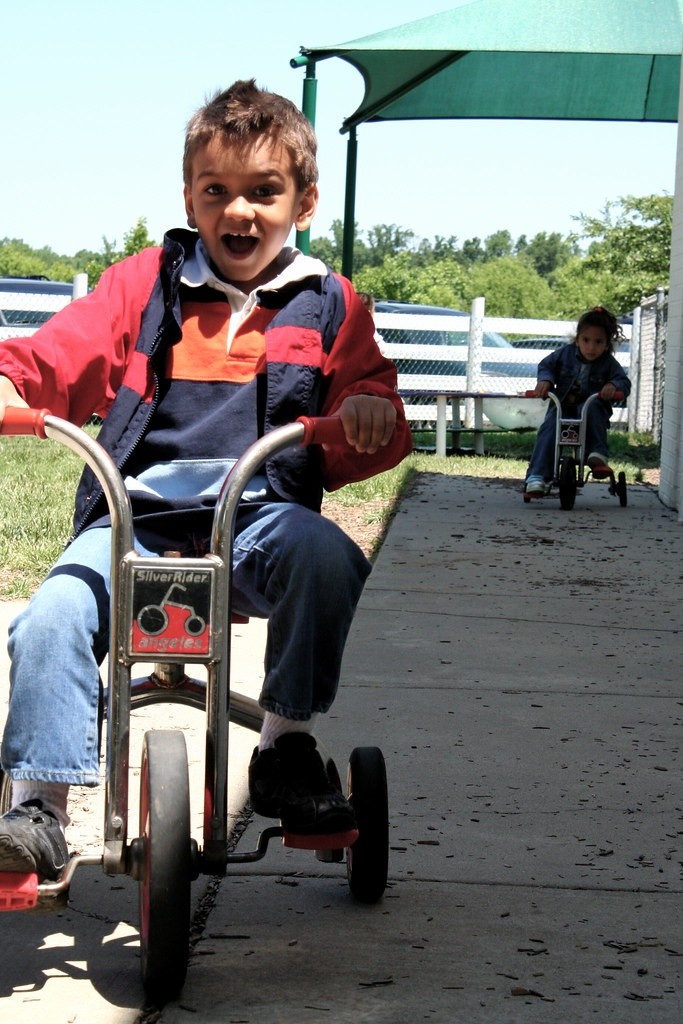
[0,403,393,1024]
[523,388,628,511]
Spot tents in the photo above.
[290,0,683,283]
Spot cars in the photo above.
[375,302,551,431]
[510,338,630,412]
[0,278,95,328]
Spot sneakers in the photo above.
[526,476,545,493]
[249,732,359,836]
[588,453,609,479]
[0,801,71,910]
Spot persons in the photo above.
[526,306,631,497]
[1,79,412,916]
